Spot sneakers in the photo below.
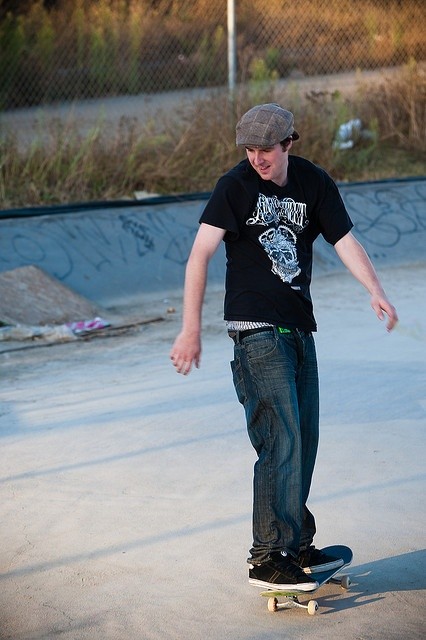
[291,545,344,574]
[247,551,319,592]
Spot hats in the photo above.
[234,102,299,146]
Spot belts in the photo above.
[232,325,293,342]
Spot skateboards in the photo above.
[260,545,352,614]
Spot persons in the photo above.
[170,102,401,592]
